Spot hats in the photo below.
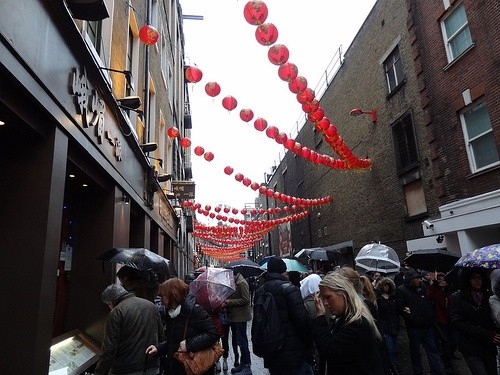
[406,271,421,278]
[267,257,287,274]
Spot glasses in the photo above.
[410,276,422,280]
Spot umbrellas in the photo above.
[105,244,500,277]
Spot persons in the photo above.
[101,258,500,375]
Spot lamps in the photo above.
[119,104,146,127]
[101,67,134,91]
[350,109,378,121]
[146,155,163,168]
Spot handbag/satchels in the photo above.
[173,303,224,375]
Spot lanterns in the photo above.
[141,0,372,258]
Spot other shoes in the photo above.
[215,357,228,370]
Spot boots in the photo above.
[231,362,243,375]
[234,364,253,375]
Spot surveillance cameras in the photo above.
[424,220,434,229]
